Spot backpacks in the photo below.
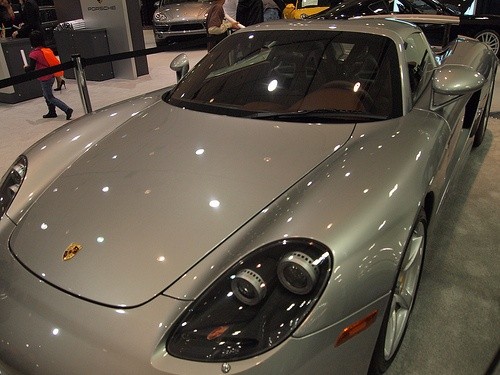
[32,48,64,77]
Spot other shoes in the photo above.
[43,113,57,118]
[66,109,73,120]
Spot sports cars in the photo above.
[0,19,500,375]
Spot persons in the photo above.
[0,0,19,39]
[12,0,66,91]
[205,0,297,53]
[23,30,73,120]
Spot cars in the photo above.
[282,0,500,59]
[153,0,222,48]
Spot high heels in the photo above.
[54,80,66,91]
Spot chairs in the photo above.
[242,37,392,115]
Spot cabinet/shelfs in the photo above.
[53,28,115,82]
